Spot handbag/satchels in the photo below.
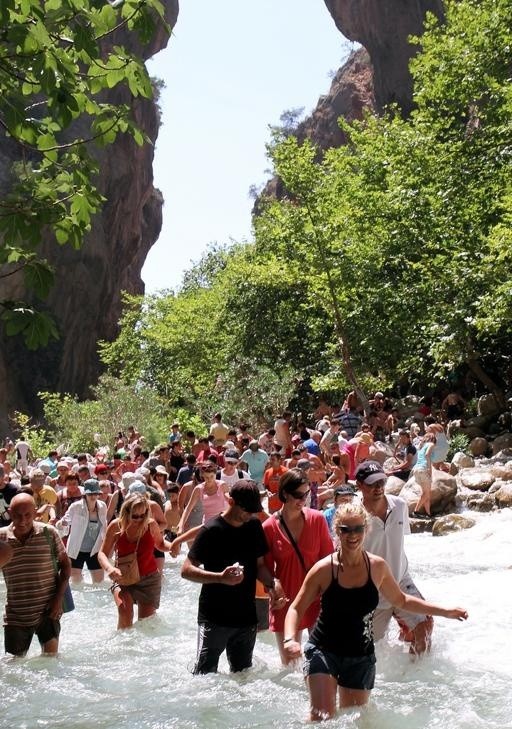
[114,553,141,587]
[56,574,74,613]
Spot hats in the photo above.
[355,460,388,486]
[334,484,357,497]
[229,480,263,513]
[224,449,240,462]
[84,479,104,494]
[118,467,150,496]
[153,465,169,480]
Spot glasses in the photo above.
[336,525,365,534]
[290,490,310,499]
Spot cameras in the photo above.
[231,562,245,576]
